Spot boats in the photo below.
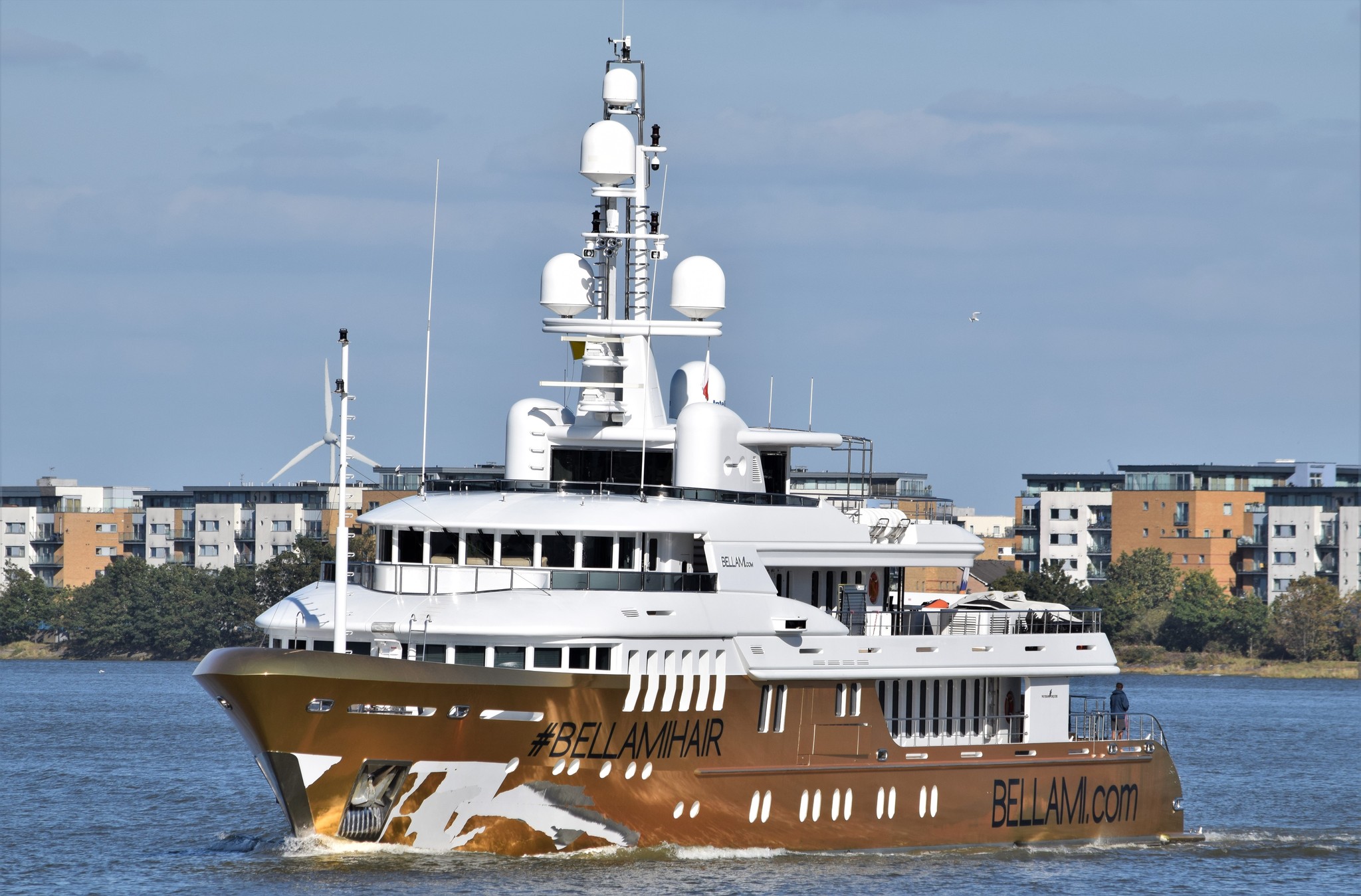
[192,28,1207,862]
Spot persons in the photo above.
[1110,682,1130,741]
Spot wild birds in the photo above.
[968,312,982,323]
[392,464,405,477]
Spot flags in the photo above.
[700,350,709,401]
